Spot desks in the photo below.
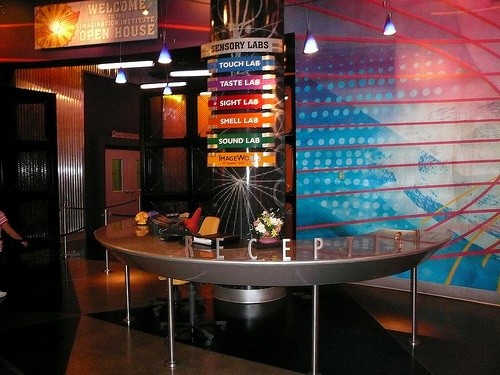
[94,219,452,375]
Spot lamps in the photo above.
[158,40,172,64]
[303,31,319,54]
[98,58,155,71]
[113,67,127,84]
[383,12,396,36]
[140,70,214,95]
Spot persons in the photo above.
[0,211,28,253]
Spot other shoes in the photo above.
[0,291,7,298]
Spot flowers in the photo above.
[253,207,285,236]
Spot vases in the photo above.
[258,235,280,246]
